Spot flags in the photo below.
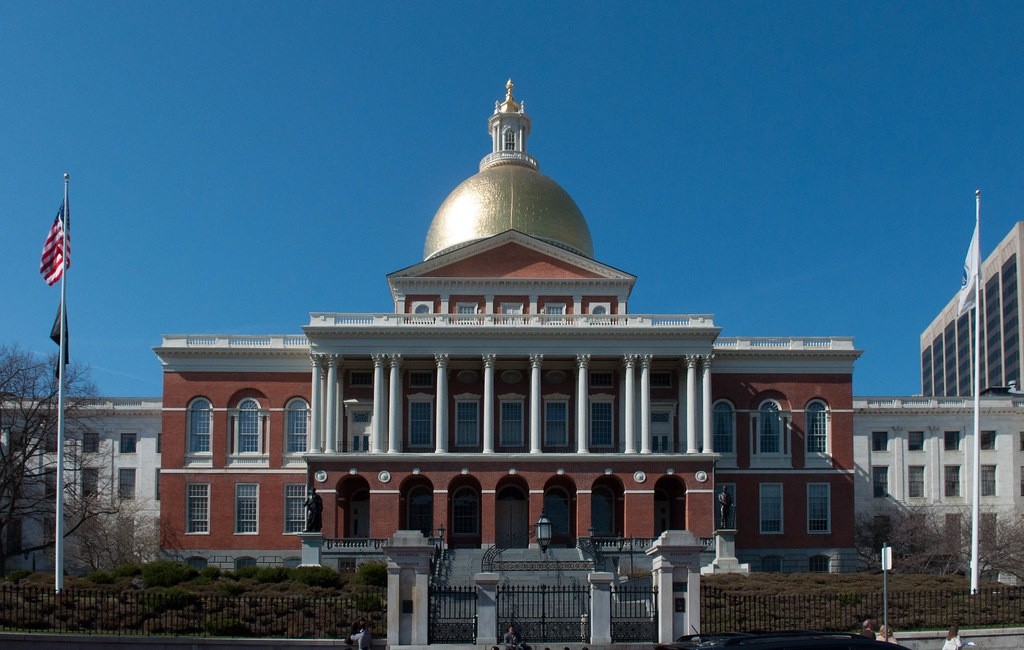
[40,197,70,287]
[956,223,984,322]
[50,300,69,380]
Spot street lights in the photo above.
[616,534,636,601]
[438,555,451,589]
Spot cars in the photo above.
[655,631,911,650]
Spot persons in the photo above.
[717,483,731,529]
[504,625,521,650]
[862,620,965,650]
[345,617,371,650]
[303,487,323,533]
